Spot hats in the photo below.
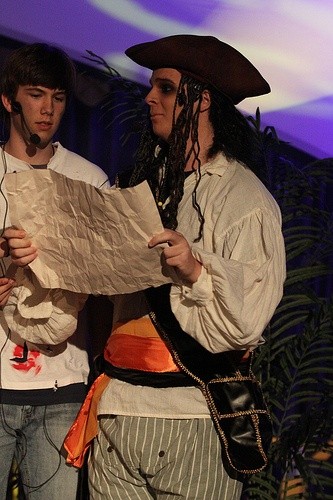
[123,34,272,106]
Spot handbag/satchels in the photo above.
[205,371,277,474]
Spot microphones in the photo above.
[11,101,42,146]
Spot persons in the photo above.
[4,27,287,500]
[0,42,118,499]
